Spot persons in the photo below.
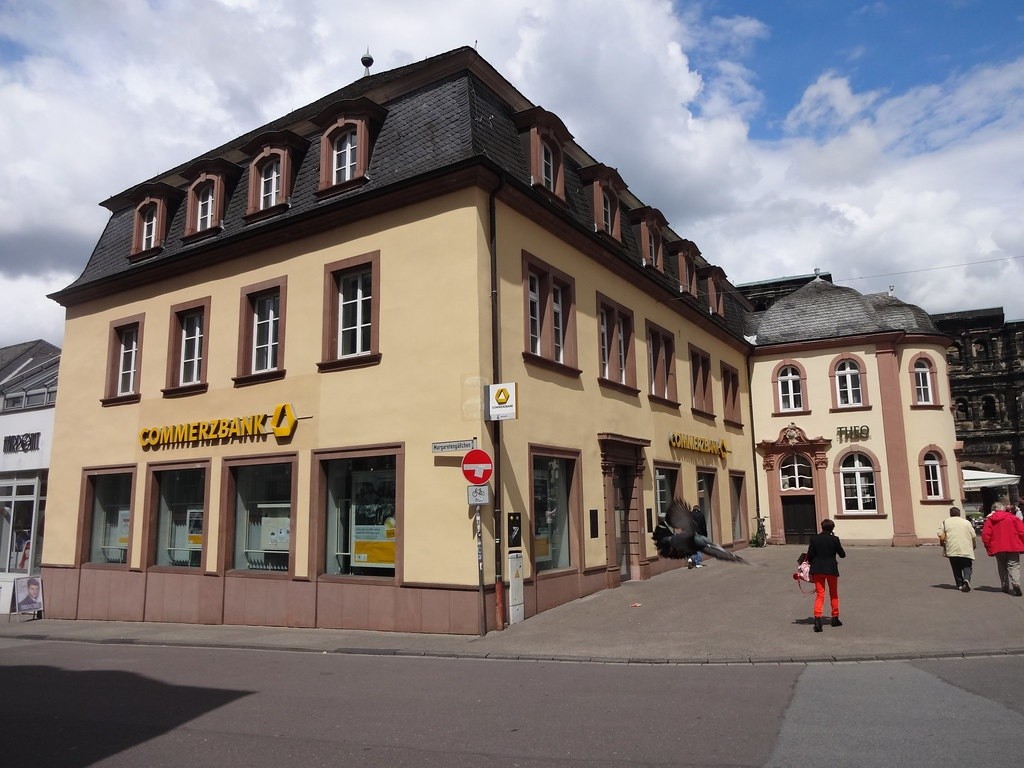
[937,507,977,593]
[683,505,708,568]
[981,502,1024,596]
[1005,497,1024,520]
[806,519,846,632]
[17,578,41,612]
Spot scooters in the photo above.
[751,516,770,547]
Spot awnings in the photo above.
[962,469,1021,489]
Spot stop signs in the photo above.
[460,449,493,485]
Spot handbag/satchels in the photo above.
[798,553,807,565]
[940,521,946,545]
[793,563,816,595]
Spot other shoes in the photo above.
[959,579,970,591]
[1013,583,1022,595]
[1002,586,1008,592]
[813,618,822,630]
[832,618,842,628]
